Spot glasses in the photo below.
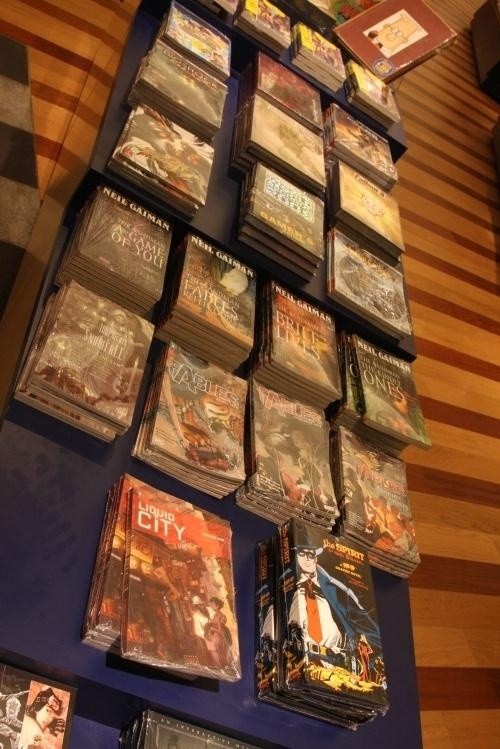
[388,24,407,40]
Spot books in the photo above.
[2,0,460,749]
